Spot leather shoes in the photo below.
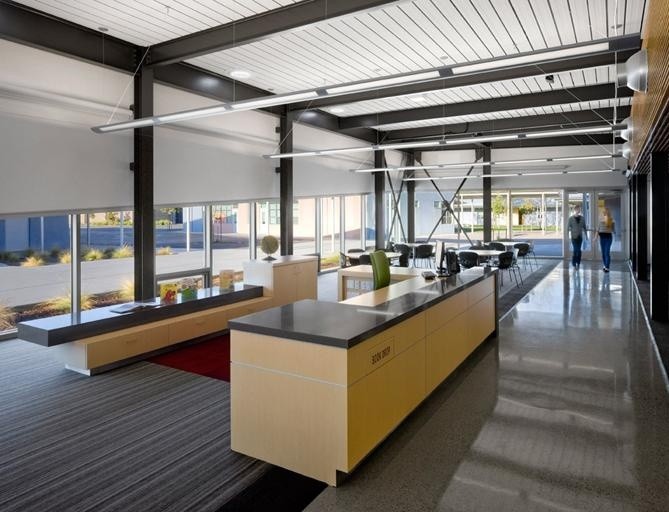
[603,266,610,273]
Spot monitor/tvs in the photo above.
[434,240,451,277]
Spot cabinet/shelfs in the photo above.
[17,255,319,377]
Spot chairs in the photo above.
[339,240,538,291]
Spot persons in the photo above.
[566,205,588,271]
[571,271,584,334]
[591,207,618,273]
[599,273,615,340]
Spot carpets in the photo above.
[0,337,268,512]
[141,327,230,383]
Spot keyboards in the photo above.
[421,271,435,278]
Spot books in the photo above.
[110,303,162,314]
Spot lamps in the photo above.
[261,119,617,161]
[398,167,627,181]
[348,153,613,174]
[89,29,647,135]
